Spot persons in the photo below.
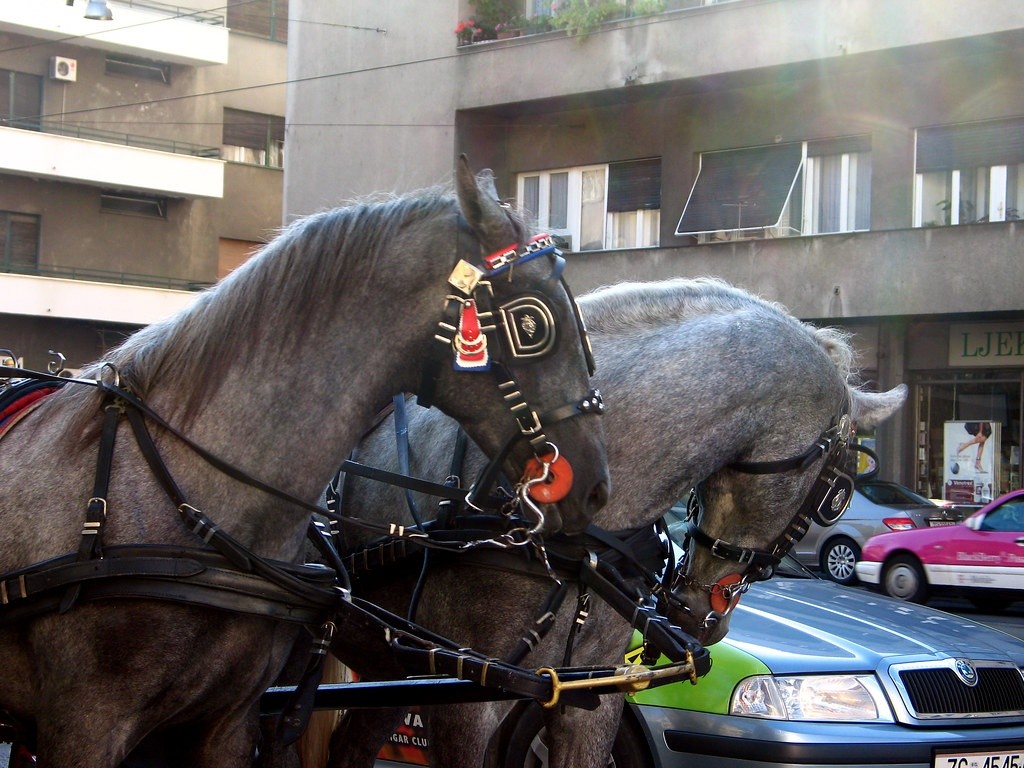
[957,422,992,473]
[998,491,1007,497]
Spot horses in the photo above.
[0,152,908,768]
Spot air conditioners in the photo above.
[48,55,77,83]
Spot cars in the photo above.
[666,474,965,589]
[853,485,1024,605]
[611,550,1024,768]
